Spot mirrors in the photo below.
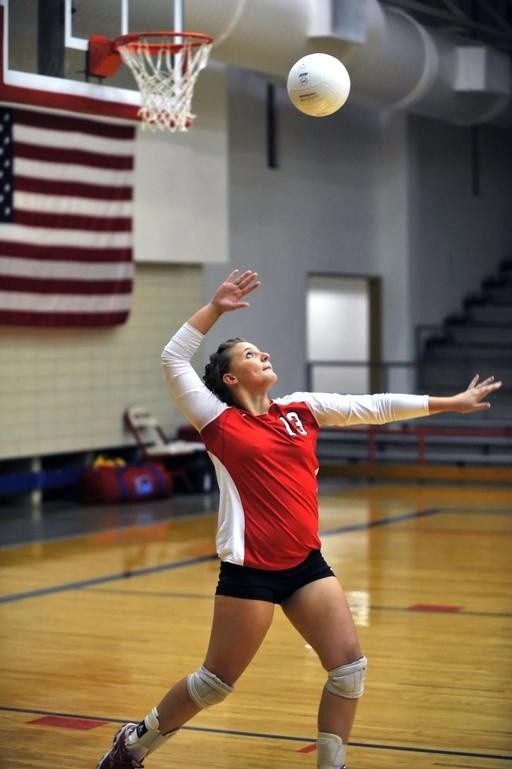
[425,335,512,368]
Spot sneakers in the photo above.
[95,723,145,769]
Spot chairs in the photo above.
[123,405,220,503]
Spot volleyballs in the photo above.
[286,50,354,119]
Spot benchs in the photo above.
[317,432,512,448]
[388,418,511,429]
[498,261,512,274]
[482,276,512,296]
[317,449,511,467]
[463,295,512,319]
[443,314,512,341]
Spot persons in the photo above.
[100,270,503,769]
